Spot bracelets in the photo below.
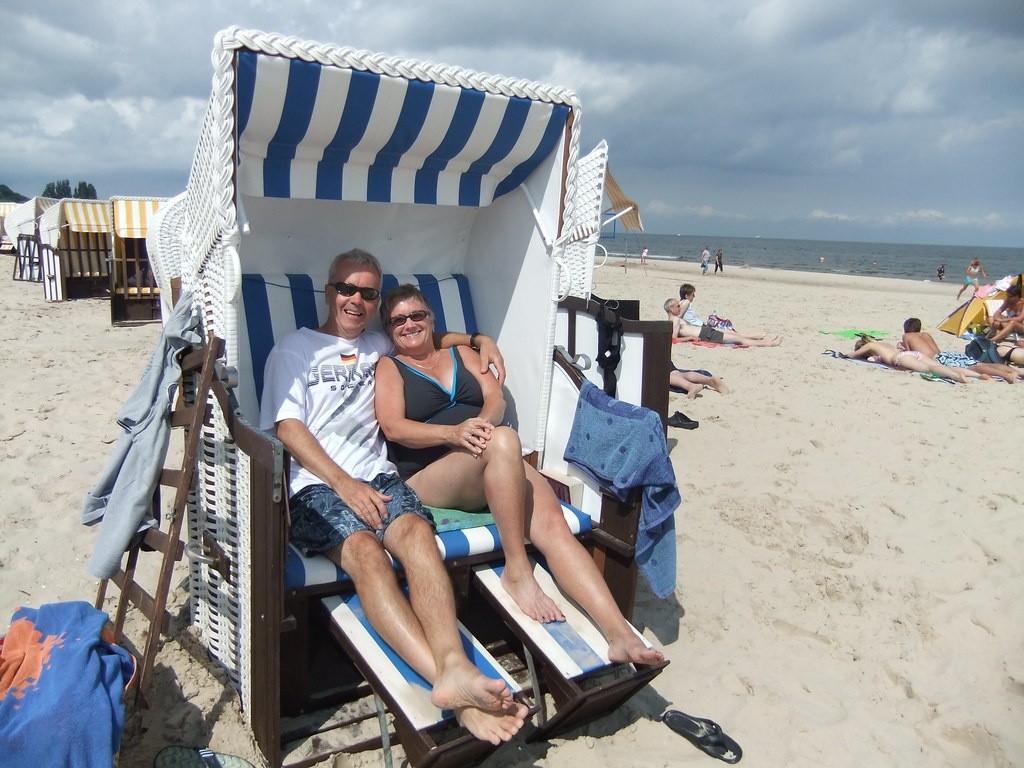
[470,333,481,349]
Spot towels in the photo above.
[0,600,135,768]
[818,328,890,340]
[832,349,1023,386]
[672,337,750,350]
[668,368,724,395]
[562,378,683,600]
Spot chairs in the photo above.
[984,299,1019,341]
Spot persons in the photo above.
[714,249,723,275]
[848,336,996,384]
[937,264,944,281]
[677,284,766,340]
[669,360,729,400]
[641,246,648,265]
[957,257,986,301]
[664,298,784,347]
[260,248,528,745]
[375,284,664,666]
[991,284,1024,365]
[702,246,711,275]
[896,318,1024,385]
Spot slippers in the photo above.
[663,710,743,765]
[821,349,835,354]
[667,411,699,429]
[154,746,255,768]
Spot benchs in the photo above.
[1,24,670,768]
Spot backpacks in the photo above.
[965,337,1005,364]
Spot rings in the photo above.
[472,429,474,436]
[468,435,472,443]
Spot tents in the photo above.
[936,273,1024,337]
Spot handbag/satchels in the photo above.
[707,314,733,328]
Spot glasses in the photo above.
[386,309,430,328]
[328,282,379,300]
[673,305,681,308]
[690,294,695,297]
